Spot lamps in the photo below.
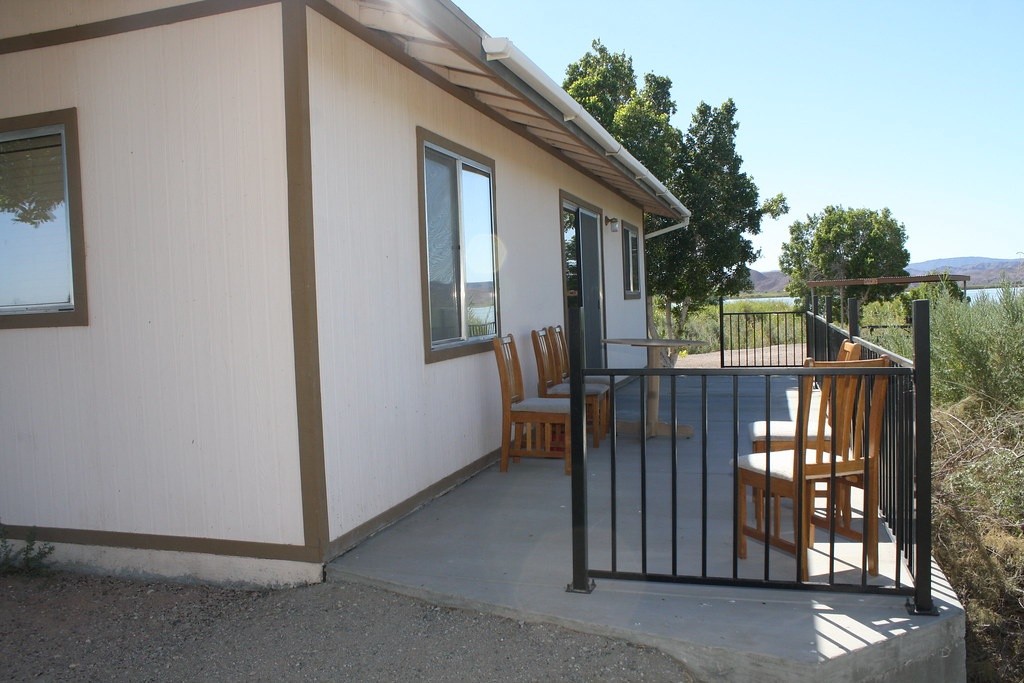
[605,216,619,232]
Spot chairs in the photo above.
[531,325,610,448]
[493,333,572,475]
[733,339,889,582]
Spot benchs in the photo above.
[863,325,911,342]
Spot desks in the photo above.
[602,338,710,440]
